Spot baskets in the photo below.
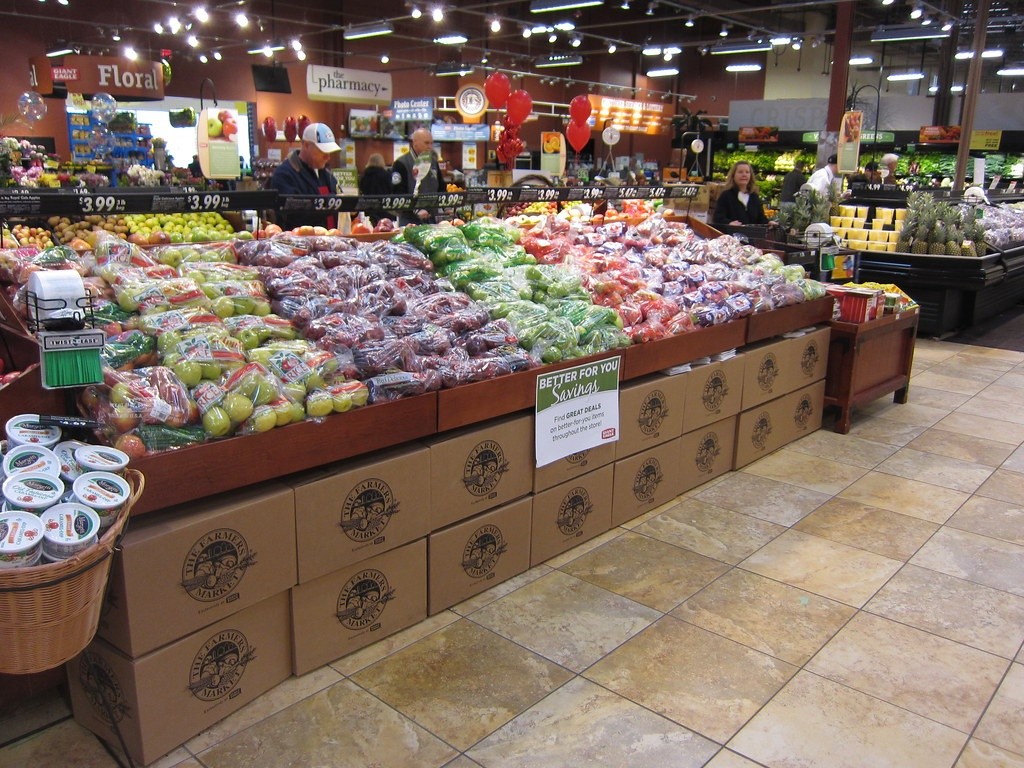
[0,439,145,674]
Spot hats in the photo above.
[827,154,837,163]
[302,123,342,154]
[865,162,877,171]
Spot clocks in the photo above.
[455,83,489,118]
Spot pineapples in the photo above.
[766,178,838,244]
[895,191,986,257]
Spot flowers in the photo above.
[0,136,221,191]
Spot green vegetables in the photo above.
[714,147,816,201]
[859,151,1024,179]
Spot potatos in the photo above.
[47,214,130,243]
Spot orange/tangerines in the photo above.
[544,136,559,153]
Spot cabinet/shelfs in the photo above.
[66,110,155,188]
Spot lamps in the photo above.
[831,0,1024,92]
[46,0,825,103]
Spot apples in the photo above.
[2,224,54,251]
[500,200,583,219]
[123,211,254,243]
[236,234,532,403]
[251,218,394,237]
[389,209,825,364]
[208,110,238,140]
[621,199,650,215]
[0,242,369,457]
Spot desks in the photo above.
[823,306,921,434]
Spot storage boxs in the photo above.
[0,324,69,442]
[76,323,831,768]
[664,182,726,223]
[826,282,902,323]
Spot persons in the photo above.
[806,154,852,201]
[712,161,779,232]
[359,153,390,195]
[264,123,344,231]
[847,162,882,189]
[780,160,806,213]
[390,127,447,224]
